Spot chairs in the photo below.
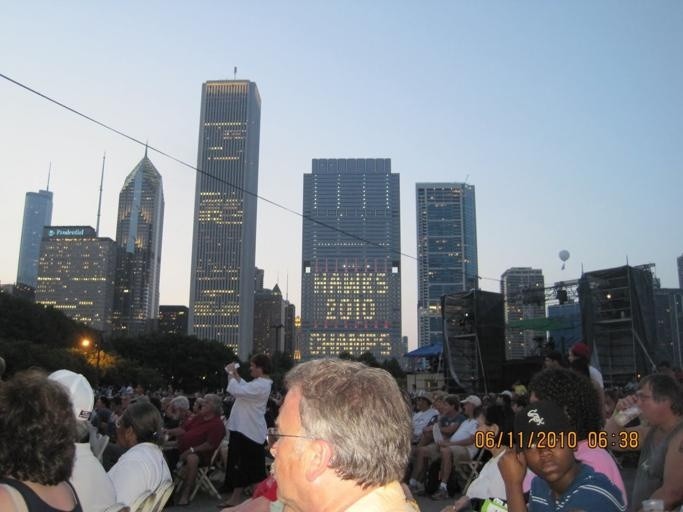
[455,443,484,493]
[189,442,228,502]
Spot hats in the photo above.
[461,395,481,407]
[417,390,433,404]
[572,342,589,359]
[48,369,94,420]
[514,400,567,453]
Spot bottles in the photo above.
[164,431,169,444]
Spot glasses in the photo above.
[264,427,307,446]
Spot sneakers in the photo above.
[409,481,425,497]
[432,485,449,500]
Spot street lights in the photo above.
[269,323,284,352]
[80,337,101,366]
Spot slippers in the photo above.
[217,501,234,508]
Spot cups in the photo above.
[614,404,641,428]
[641,498,665,512]
